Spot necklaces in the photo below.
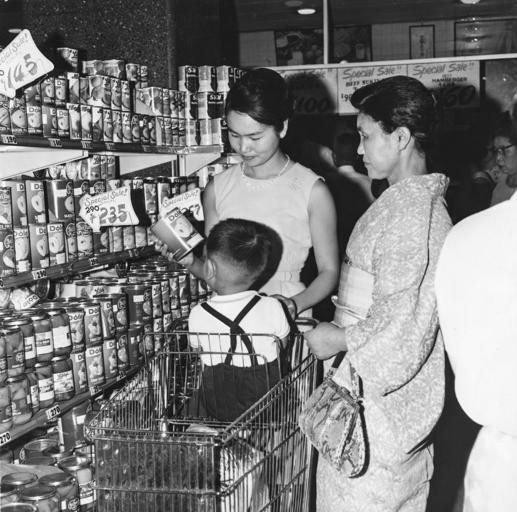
[238,152,291,191]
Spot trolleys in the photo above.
[81,314,321,512]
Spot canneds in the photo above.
[0,47,246,512]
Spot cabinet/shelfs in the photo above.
[0,133,222,450]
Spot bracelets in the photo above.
[290,298,298,318]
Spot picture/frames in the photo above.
[409,25,435,58]
[454,19,517,56]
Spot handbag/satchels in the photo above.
[297,378,369,479]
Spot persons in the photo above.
[187,217,296,424]
[486,116,517,207]
[313,131,378,260]
[153,65,341,512]
[433,186,517,511]
[296,72,455,512]
